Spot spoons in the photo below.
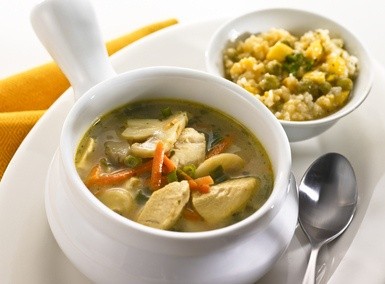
[295,151,360,284]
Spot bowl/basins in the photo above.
[204,8,374,142]
[25,1,301,282]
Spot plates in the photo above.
[0,8,385,283]
